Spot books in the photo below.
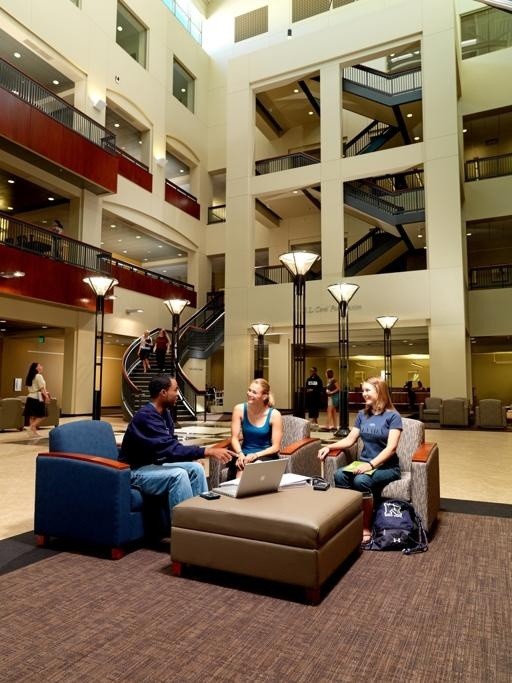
[37,392,51,402]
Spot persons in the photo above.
[49,219,64,260]
[117,372,239,510]
[153,329,170,371]
[137,329,154,372]
[226,378,284,478]
[304,366,324,424]
[20,362,51,436]
[316,377,403,544]
[319,370,340,432]
[415,380,426,392]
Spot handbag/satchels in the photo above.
[332,393,339,407]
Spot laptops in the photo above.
[214,459,289,499]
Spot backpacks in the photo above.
[372,499,418,548]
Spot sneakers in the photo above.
[26,427,41,437]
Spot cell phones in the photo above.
[312,479,331,491]
[199,490,220,501]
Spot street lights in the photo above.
[251,321,268,377]
[375,313,399,403]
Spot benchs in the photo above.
[348,387,430,410]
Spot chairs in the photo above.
[323,416,436,535]
[418,397,512,429]
[208,414,322,488]
[0,392,59,431]
[34,420,167,560]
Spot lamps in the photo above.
[252,324,271,379]
[375,315,398,396]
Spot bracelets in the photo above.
[253,452,259,459]
[368,462,375,470]
